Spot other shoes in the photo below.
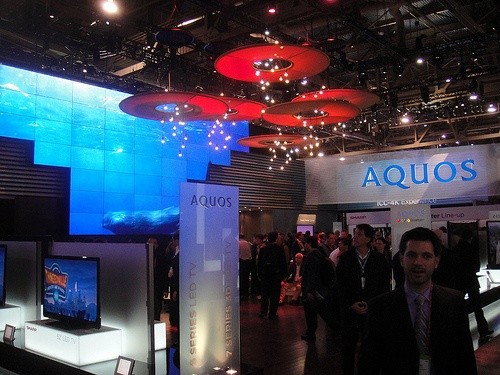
[301,334,316,341]
[480,330,494,338]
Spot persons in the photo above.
[147,236,179,327]
[343,224,390,375]
[431,226,494,337]
[372,228,392,290]
[356,226,478,375]
[300,237,336,343]
[239,230,307,320]
[304,229,354,267]
[392,250,406,290]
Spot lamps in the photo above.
[487,103,496,112]
[469,92,478,100]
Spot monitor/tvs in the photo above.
[41,255,101,331]
[0,244,8,307]
[296,225,314,236]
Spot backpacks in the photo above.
[305,253,337,284]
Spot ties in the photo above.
[414,295,430,356]
